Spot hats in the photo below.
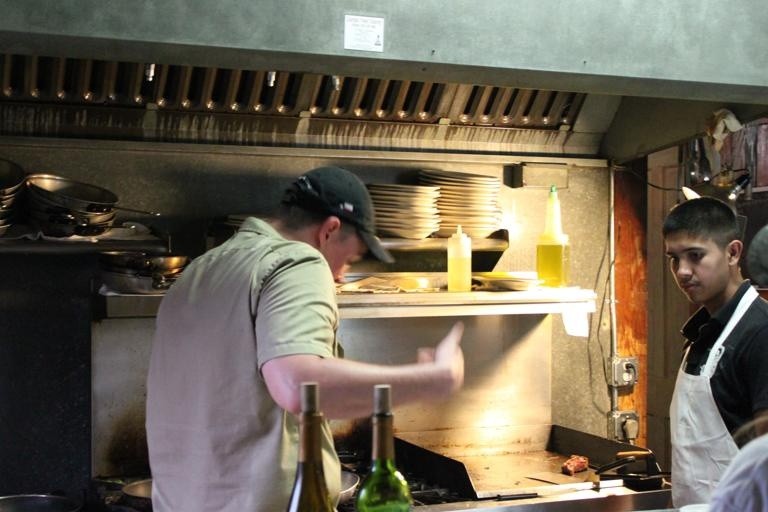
[280,162,400,267]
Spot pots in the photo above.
[30,174,162,219]
[123,476,164,506]
[98,248,185,294]
[0,164,27,235]
[26,181,117,223]
[25,212,116,237]
[329,460,360,507]
[2,493,82,512]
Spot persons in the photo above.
[706,223,768,512]
[144,167,464,511]
[663,197,768,511]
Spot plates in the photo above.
[425,171,504,239]
[368,176,440,242]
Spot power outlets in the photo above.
[607,410,639,441]
[609,360,639,388]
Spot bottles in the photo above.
[354,391,415,511]
[285,380,333,512]
